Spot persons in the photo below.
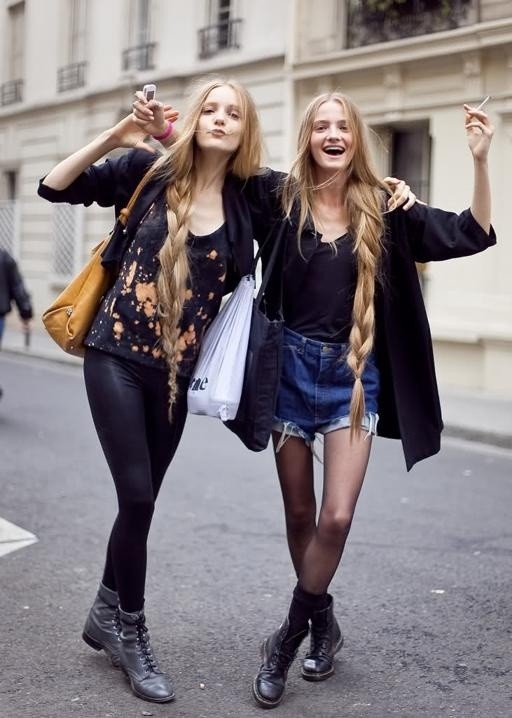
[1,245,34,398]
[123,78,500,711]
[33,71,428,705]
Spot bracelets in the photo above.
[152,120,174,141]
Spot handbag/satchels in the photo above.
[185,200,300,452]
[41,154,168,358]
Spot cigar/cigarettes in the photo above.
[476,95,490,111]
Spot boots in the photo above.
[81,581,122,667]
[117,602,175,703]
[300,593,344,682]
[253,615,312,709]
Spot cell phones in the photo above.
[142,84,156,102]
